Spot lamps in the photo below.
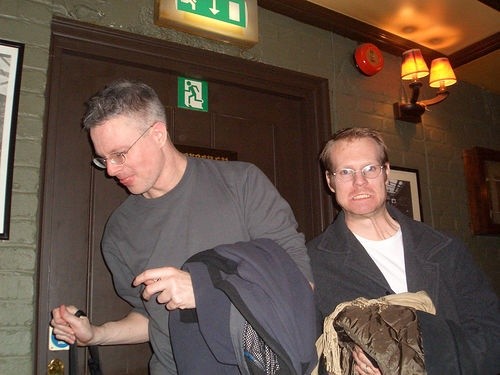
[393,49,457,123]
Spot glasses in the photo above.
[93,122,155,169]
[327,164,385,181]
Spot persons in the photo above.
[51,77,315,375]
[307,127,500,375]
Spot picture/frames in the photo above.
[0,39,25,240]
[463,145,500,237]
[386,166,424,223]
